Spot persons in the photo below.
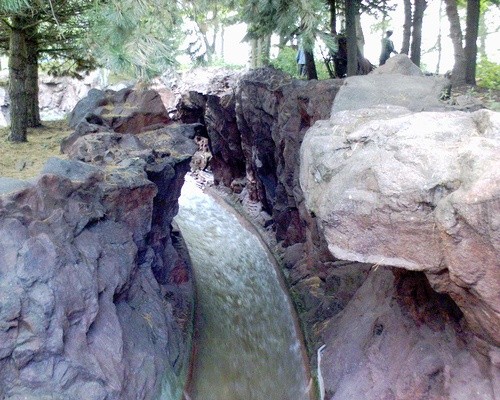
[380,30,398,65]
[297,45,308,76]
